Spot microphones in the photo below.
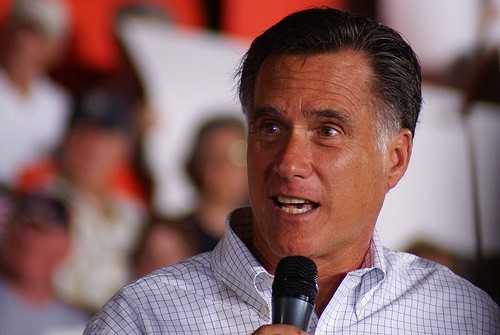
[271,256,318,331]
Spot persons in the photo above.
[0,0,257,335]
[81,6,499,335]
